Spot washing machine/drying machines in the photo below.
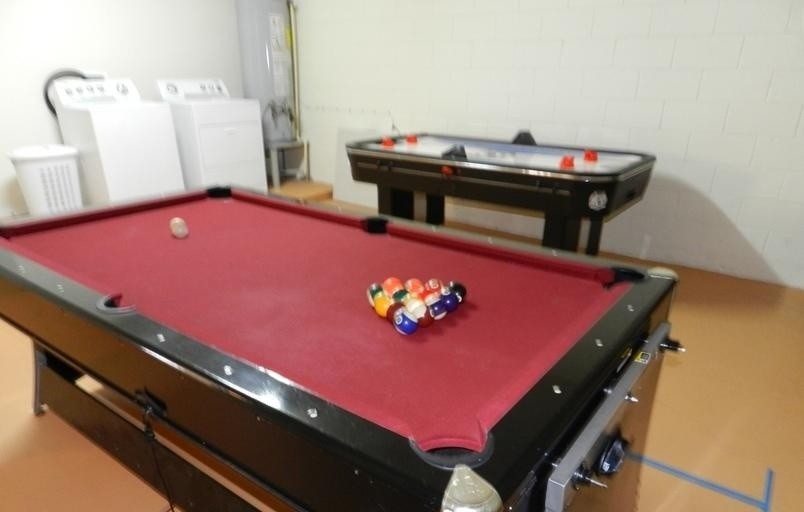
[54,79,185,204]
[138,79,269,195]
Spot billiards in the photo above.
[170,218,188,238]
[367,277,466,335]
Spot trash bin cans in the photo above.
[7,143,83,218]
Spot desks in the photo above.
[344,121,658,257]
[1,181,684,512]
